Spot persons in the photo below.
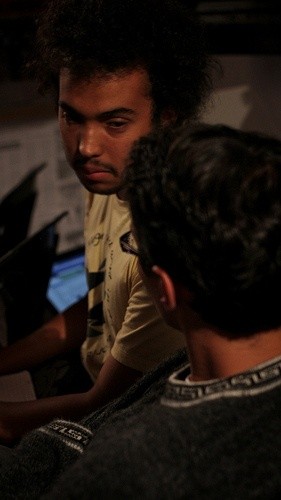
[0,1,215,450]
[0,125,281,499]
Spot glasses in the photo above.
[120,231,153,266]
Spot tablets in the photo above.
[46,245,86,314]
